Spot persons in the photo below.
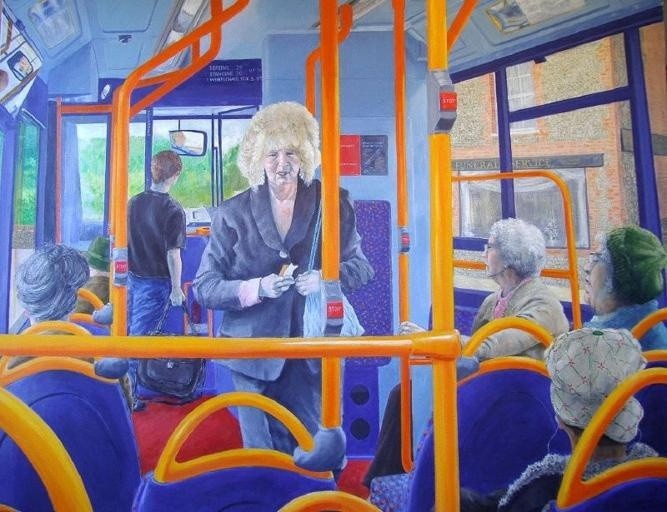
[395,218,567,368]
[123,150,188,410]
[12,241,105,342]
[190,97,374,454]
[455,321,662,512]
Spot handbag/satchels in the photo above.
[302,273,365,372]
[138,299,207,397]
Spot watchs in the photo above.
[578,225,666,352]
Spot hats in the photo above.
[543,326,646,442]
[605,226,667,304]
[82,236,111,269]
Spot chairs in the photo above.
[374,302,665,510]
[0,289,380,510]
